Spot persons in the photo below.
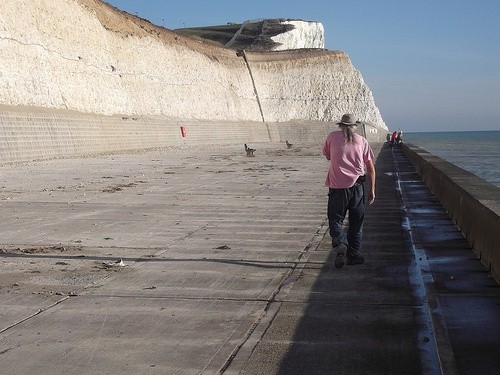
[397,131,403,143]
[390,131,398,150]
[322,113,376,267]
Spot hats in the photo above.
[335,113,357,126]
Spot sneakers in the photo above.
[346,254,365,265]
[335,243,347,269]
